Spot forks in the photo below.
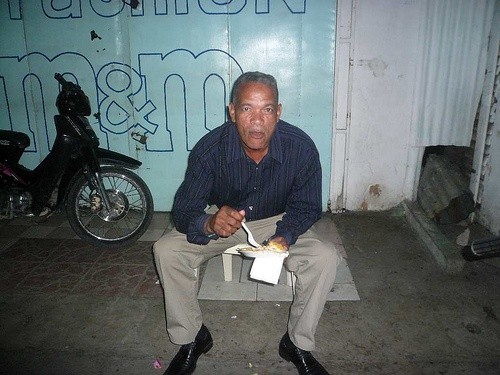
[241,220,262,248]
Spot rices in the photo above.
[240,241,286,252]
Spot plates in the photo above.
[238,246,289,258]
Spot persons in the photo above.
[153,71,337,375]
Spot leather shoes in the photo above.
[164,324,213,375]
[279,331,329,375]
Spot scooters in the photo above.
[0,71,155,245]
